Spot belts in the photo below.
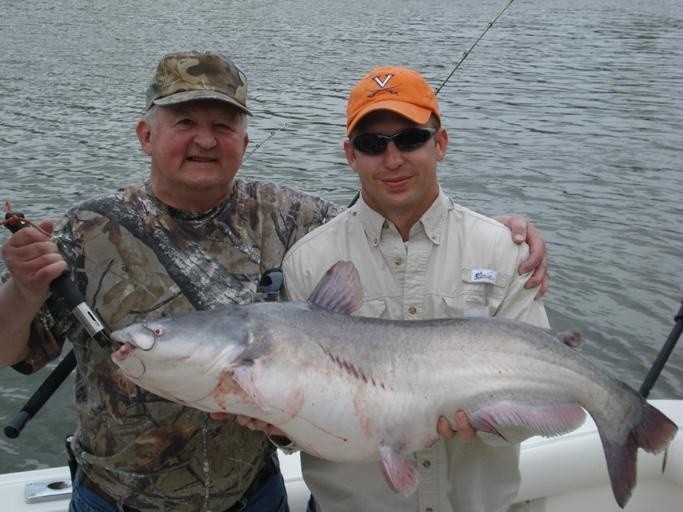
[81,466,270,512]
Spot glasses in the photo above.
[353,127,438,155]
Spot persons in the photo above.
[0,47,550,511]
[210,67,549,512]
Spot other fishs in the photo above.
[110,260,680,508]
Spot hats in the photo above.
[346,65,441,136]
[146,51,253,117]
